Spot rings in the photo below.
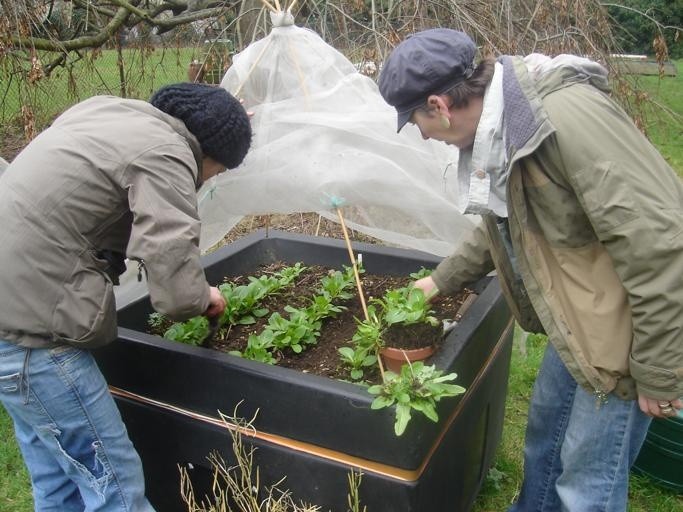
[659,402,673,413]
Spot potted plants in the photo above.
[376,284,443,373]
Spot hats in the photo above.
[149,81,253,170]
[376,27,479,135]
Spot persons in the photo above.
[0,82,256,512]
[378,28,683,512]
